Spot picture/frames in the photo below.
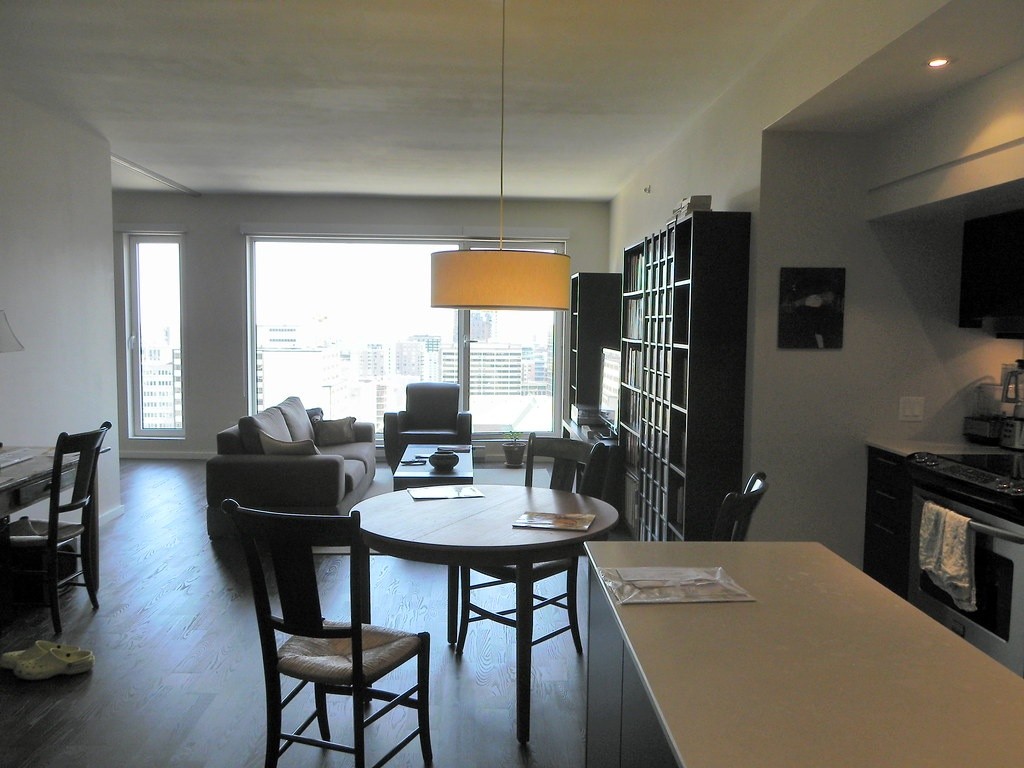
[778,265,847,351]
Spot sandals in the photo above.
[14,648,94,680]
[0,640,81,670]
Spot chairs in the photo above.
[4,421,114,637]
[710,471,770,544]
[219,497,435,768]
[456,432,605,661]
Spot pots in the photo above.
[978,382,1016,418]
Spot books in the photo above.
[438,447,470,452]
[512,511,595,531]
[407,487,484,498]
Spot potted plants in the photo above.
[502,431,526,468]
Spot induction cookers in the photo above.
[912,454,1024,525]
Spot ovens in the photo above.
[904,485,1023,681]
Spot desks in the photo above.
[0,444,111,596]
[347,484,620,744]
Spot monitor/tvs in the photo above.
[599,347,622,439]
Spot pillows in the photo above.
[258,429,322,454]
[275,396,314,442]
[314,416,356,445]
[238,407,293,454]
[306,406,323,422]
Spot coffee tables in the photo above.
[392,444,475,492]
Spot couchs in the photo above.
[206,419,374,548]
[383,381,472,476]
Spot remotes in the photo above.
[401,460,426,463]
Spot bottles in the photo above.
[428,451,459,471]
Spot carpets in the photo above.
[310,468,551,554]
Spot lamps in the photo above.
[428,0,579,314]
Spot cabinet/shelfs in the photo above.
[614,212,749,542]
[559,418,619,532]
[567,272,622,424]
[585,552,680,768]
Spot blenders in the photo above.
[999,358,1024,452]
